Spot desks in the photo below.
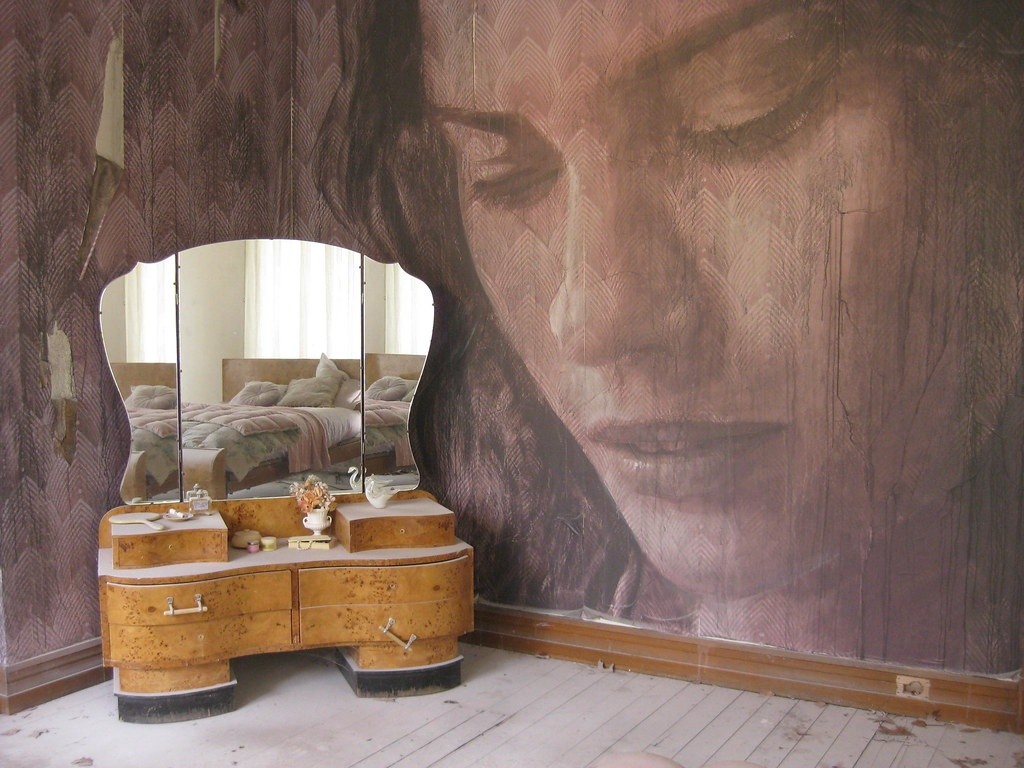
[98,488,474,725]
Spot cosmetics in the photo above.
[247,539,260,554]
[260,536,277,552]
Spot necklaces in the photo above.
[296,540,314,551]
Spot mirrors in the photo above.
[99,238,435,510]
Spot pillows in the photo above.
[277,377,341,407]
[367,376,408,402]
[231,380,280,406]
[316,354,363,410]
[130,385,177,408]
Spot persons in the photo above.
[304,1,1024,684]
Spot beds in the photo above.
[362,378,422,492]
[178,406,363,497]
[123,383,179,506]
